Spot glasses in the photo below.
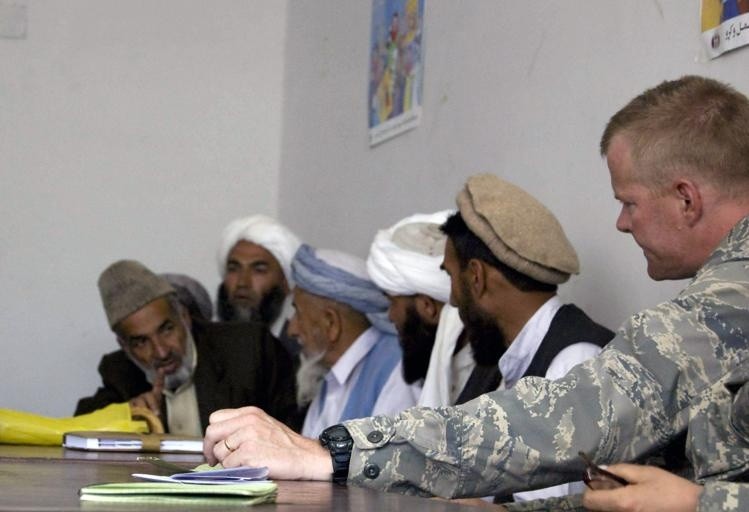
[577,451,630,490]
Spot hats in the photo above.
[97,259,177,330]
[456,174,580,285]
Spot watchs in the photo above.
[319,424,354,486]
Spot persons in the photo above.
[73,259,311,436]
[291,244,402,440]
[203,76,748,511]
[217,214,302,357]
[583,464,748,511]
[366,209,490,417]
[440,175,616,504]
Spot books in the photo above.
[63,430,204,454]
[78,481,278,496]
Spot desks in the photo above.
[2,433,487,511]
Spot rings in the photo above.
[225,440,235,452]
